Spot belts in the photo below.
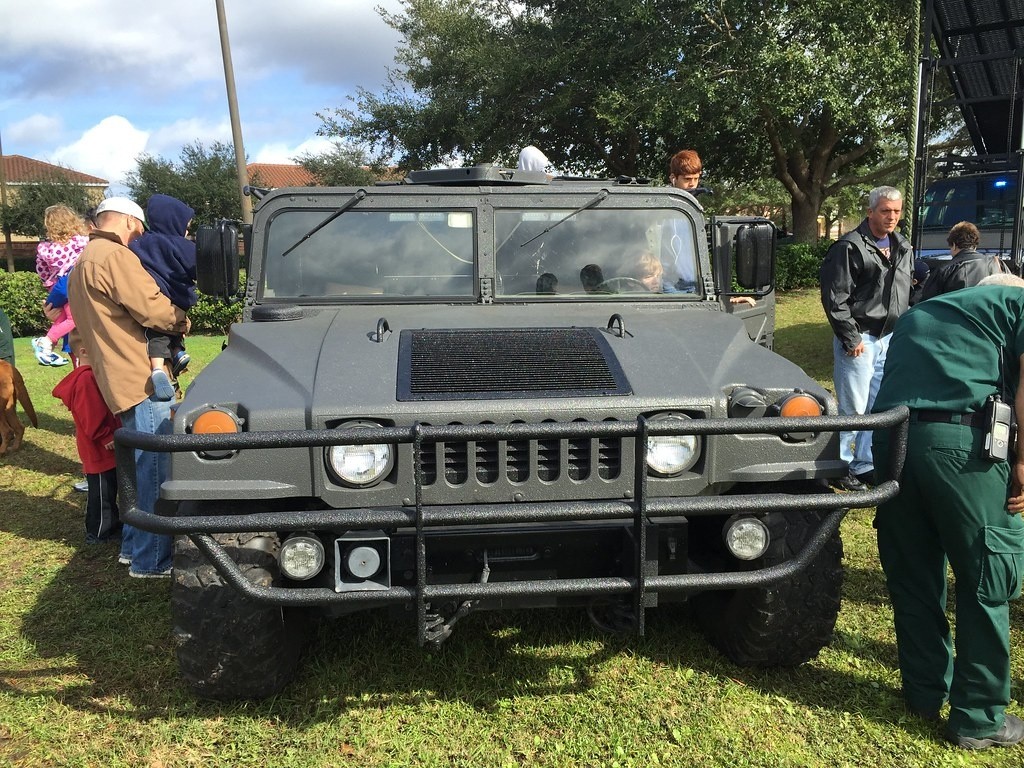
[918,410,985,428]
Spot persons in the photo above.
[909,222,1012,308]
[820,186,915,491]
[32,194,197,578]
[872,285,1024,751]
[623,253,756,308]
[0,309,15,367]
[660,150,701,291]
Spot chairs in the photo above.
[315,245,384,297]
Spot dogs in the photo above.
[0,359,38,457]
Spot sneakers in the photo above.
[172,350,190,377]
[31,336,69,367]
[150,368,176,402]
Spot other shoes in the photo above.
[827,470,874,491]
[74,481,88,491]
[914,710,941,722]
[943,712,1023,749]
[119,554,133,565]
[128,567,172,578]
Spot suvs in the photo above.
[114,164,910,696]
[897,170,1024,262]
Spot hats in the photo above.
[95,197,150,232]
